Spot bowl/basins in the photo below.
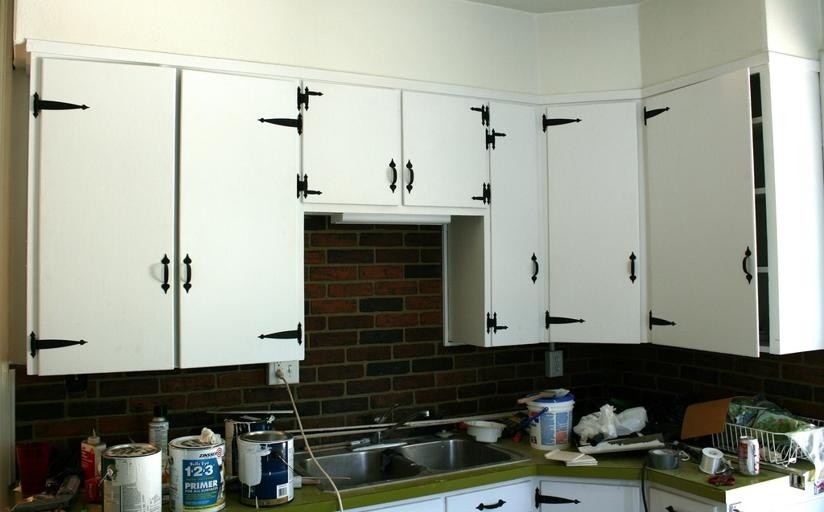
[464,419,506,444]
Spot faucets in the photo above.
[374,402,430,441]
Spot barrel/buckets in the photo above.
[101,443,162,512]
[237,430,295,507]
[170,435,227,512]
[527,392,575,452]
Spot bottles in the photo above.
[149,405,169,470]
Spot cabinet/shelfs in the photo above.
[336,461,537,512]
[441,83,546,348]
[535,466,645,511]
[644,466,726,512]
[302,79,488,226]
[548,85,643,344]
[9,35,304,375]
[645,48,821,363]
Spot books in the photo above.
[543,448,585,462]
[565,454,596,466]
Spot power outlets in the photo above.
[264,360,301,388]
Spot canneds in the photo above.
[739,436,759,475]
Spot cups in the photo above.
[698,447,729,475]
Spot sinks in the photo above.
[293,442,423,494]
[399,431,531,471]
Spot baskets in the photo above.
[711,405,824,466]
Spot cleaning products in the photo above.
[147,404,170,486]
